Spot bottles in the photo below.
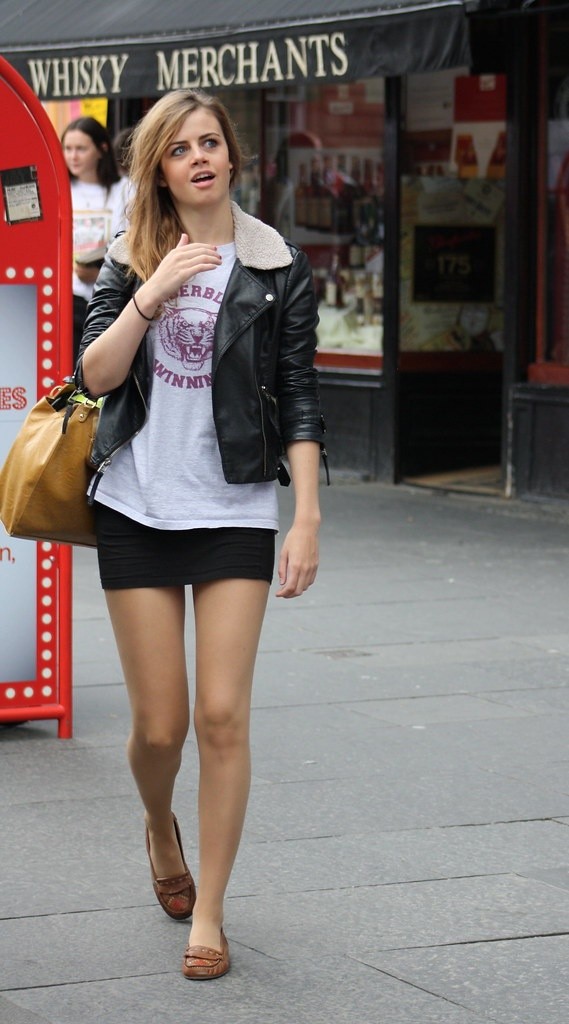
[289,125,509,318]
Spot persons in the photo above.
[107,128,136,239]
[60,117,120,371]
[71,90,326,981]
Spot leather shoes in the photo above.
[183,926,231,980]
[145,812,197,920]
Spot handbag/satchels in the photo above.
[1,353,97,550]
